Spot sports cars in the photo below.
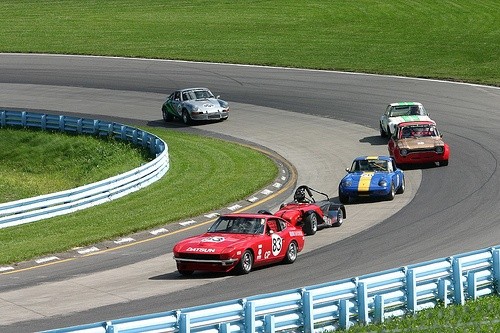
[379,101,436,140]
[388,121,450,166]
[161,88,230,125]
[338,155,405,204]
[173,213,305,276]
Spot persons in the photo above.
[401,127,417,139]
[253,219,264,234]
[410,107,420,115]
[359,160,373,171]
[294,190,310,204]
[197,92,206,99]
